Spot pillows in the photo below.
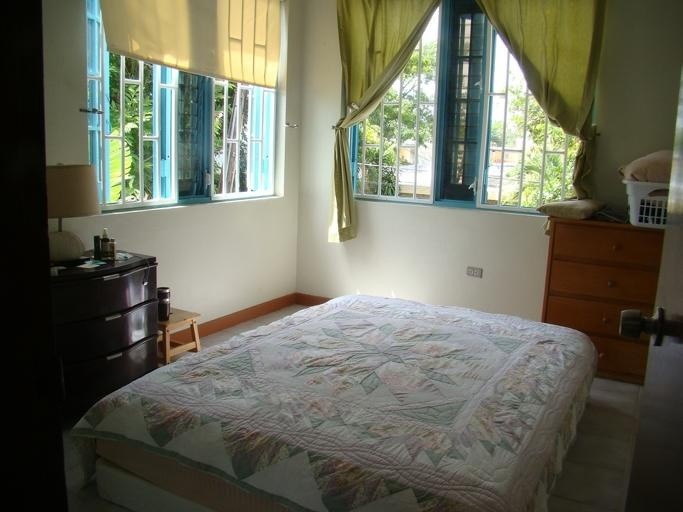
[534,199,608,218]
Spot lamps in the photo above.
[45,164,102,262]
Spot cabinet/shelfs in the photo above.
[539,220,667,387]
[50,250,159,420]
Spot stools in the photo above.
[154,308,201,364]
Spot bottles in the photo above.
[93,228,116,261]
[158,287,171,322]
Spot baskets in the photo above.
[621,179,669,229]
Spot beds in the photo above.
[83,290,598,510]
